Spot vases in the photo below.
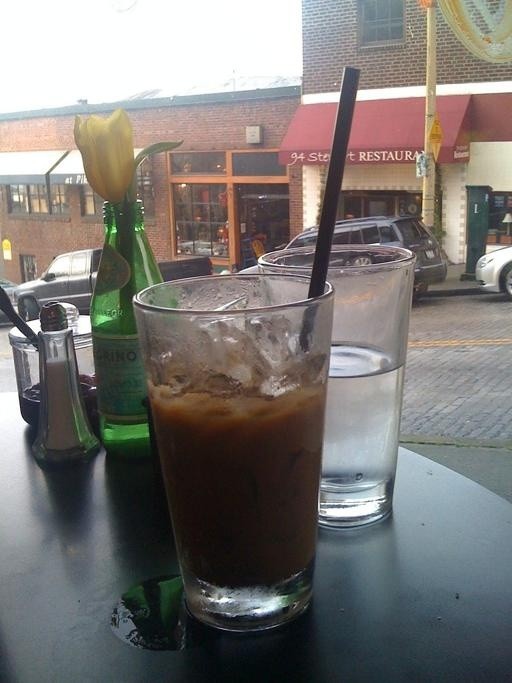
[89,198,178,461]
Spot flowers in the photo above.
[73,108,184,203]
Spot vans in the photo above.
[234,215,448,302]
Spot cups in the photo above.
[133,275,336,633]
[9,314,101,432]
[257,245,423,532]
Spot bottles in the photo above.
[90,199,165,459]
[32,301,102,465]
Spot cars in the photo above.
[0,275,18,326]
[474,245,512,300]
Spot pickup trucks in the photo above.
[12,248,212,322]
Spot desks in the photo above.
[1,388,512,683]
